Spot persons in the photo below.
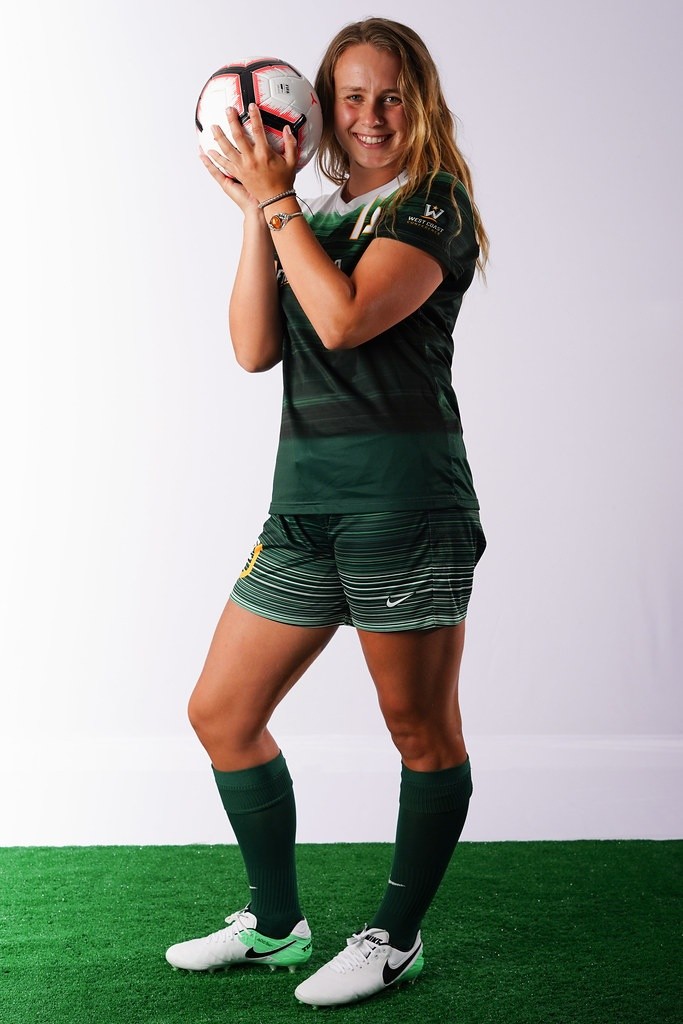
[165,18,489,1007]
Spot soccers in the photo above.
[194,54,324,185]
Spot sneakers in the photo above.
[293,921,426,1012]
[164,900,314,976]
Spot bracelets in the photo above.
[268,212,303,231]
[258,188,296,209]
